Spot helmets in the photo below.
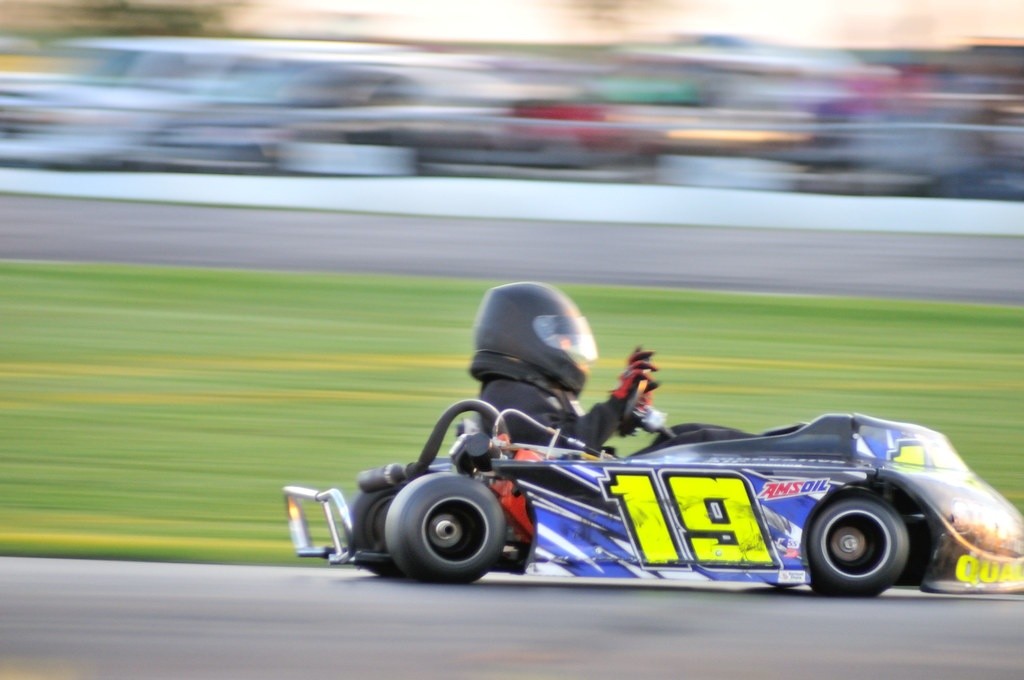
[468,281,600,398]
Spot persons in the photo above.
[470,281,662,455]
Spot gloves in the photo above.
[615,345,663,402]
